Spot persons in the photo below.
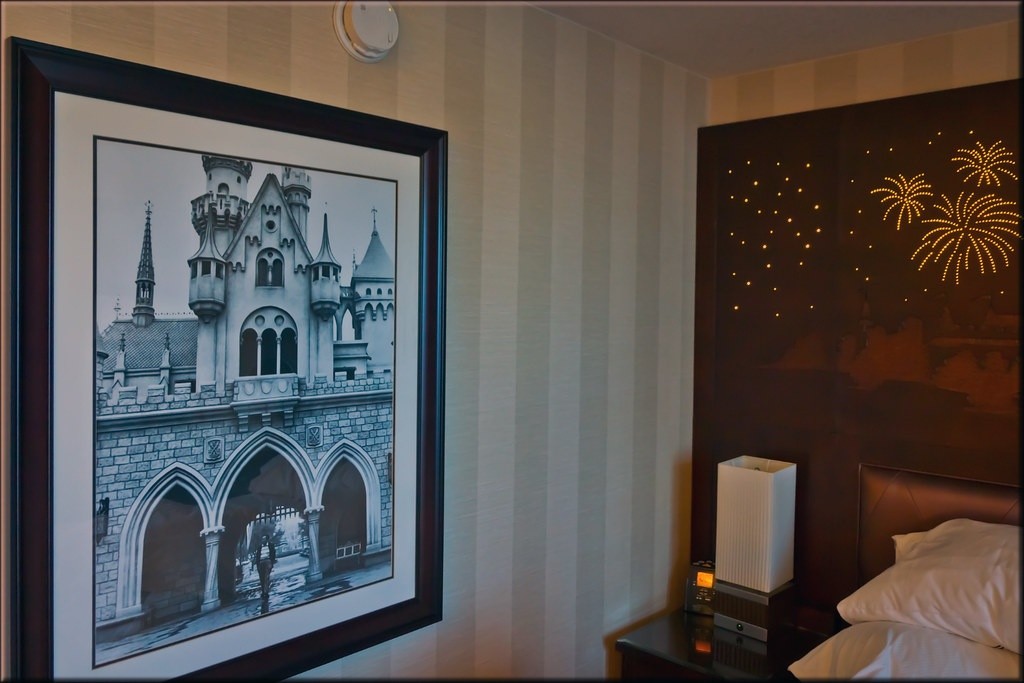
[256,535,275,599]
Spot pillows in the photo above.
[836,518,1022,656]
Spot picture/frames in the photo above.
[7,35,449,683]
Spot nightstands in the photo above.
[616,596,824,682]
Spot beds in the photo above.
[787,463,1021,679]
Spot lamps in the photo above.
[715,456,797,643]
